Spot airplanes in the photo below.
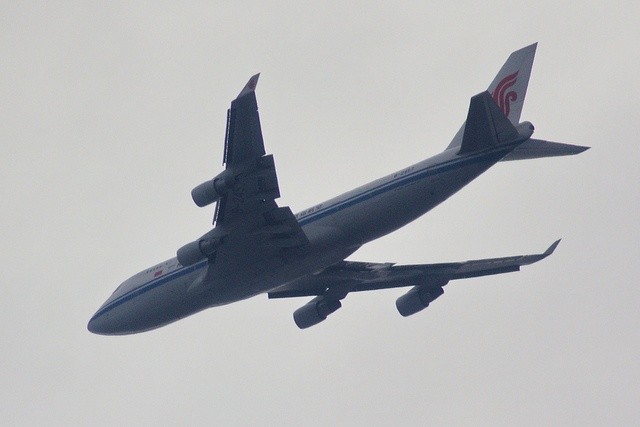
[87,42,592,335]
